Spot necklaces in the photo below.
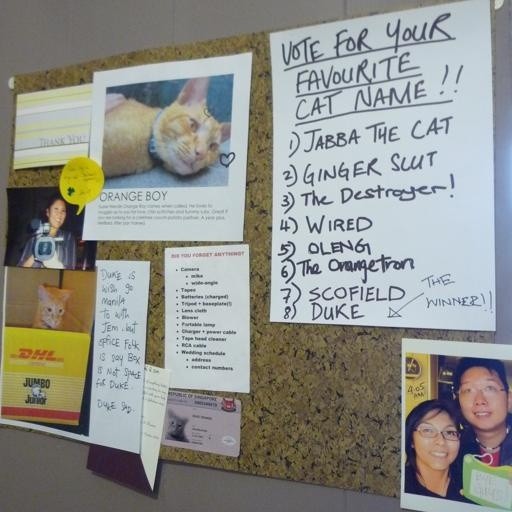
[474,437,502,450]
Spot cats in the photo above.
[102,77,232,178]
[165,409,189,442]
[32,284,73,330]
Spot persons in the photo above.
[453,356,512,507]
[14,194,77,270]
[404,397,480,504]
[30,384,42,398]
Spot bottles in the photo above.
[34,223,56,261]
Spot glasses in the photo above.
[414,424,464,440]
[459,383,508,393]
